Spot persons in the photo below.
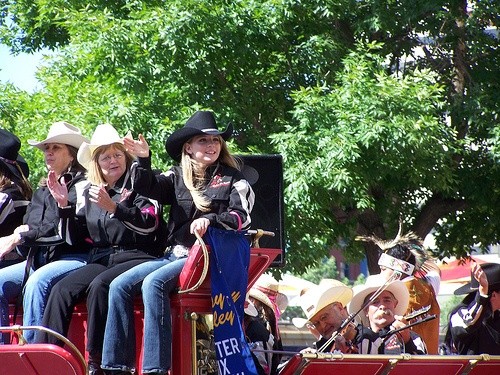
[0,121,93,344]
[98,110,256,375]
[0,129,33,267]
[35,122,168,375]
[378,244,441,355]
[292,284,385,354]
[348,275,429,355]
[241,273,288,375]
[444,262,500,357]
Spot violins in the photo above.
[329,322,360,355]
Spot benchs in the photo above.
[0,225,282,375]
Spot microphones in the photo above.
[388,314,437,335]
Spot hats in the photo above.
[249,273,288,315]
[1,129,29,180]
[27,122,89,149]
[454,263,500,295]
[165,111,234,162]
[347,275,409,330]
[77,123,133,169]
[291,280,354,329]
